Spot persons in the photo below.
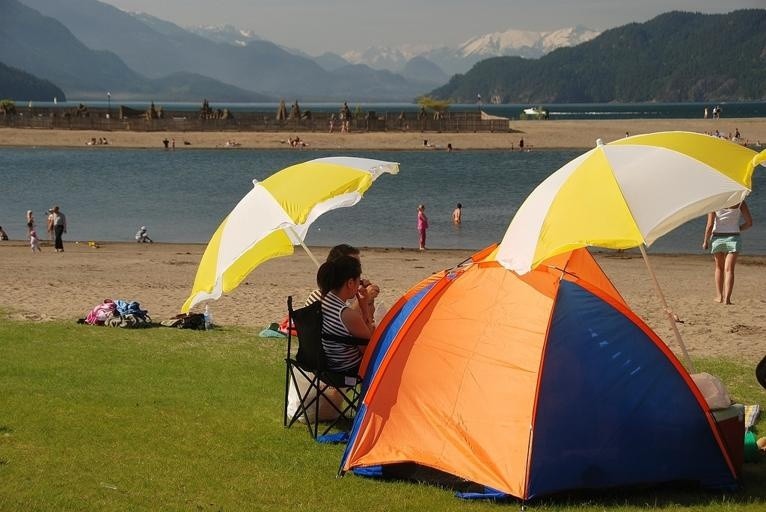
[704,128,762,146]
[85,137,107,145]
[0,226,8,240]
[713,105,721,120]
[47,205,67,252]
[172,138,176,149]
[704,107,708,119]
[163,138,169,148]
[625,132,629,136]
[227,140,241,145]
[417,205,428,251]
[519,139,524,151]
[288,136,306,148]
[305,244,380,376]
[423,139,428,146]
[702,200,753,304]
[135,226,152,243]
[452,203,462,223]
[26,210,41,252]
[448,144,452,152]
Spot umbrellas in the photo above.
[180,156,400,316]
[487,130,766,373]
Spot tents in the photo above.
[336,242,743,511]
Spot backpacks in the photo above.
[160,313,204,328]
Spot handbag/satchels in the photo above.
[288,366,342,423]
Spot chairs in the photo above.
[279,295,370,440]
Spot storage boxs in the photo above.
[707,403,745,477]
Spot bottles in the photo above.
[204,302,214,332]
